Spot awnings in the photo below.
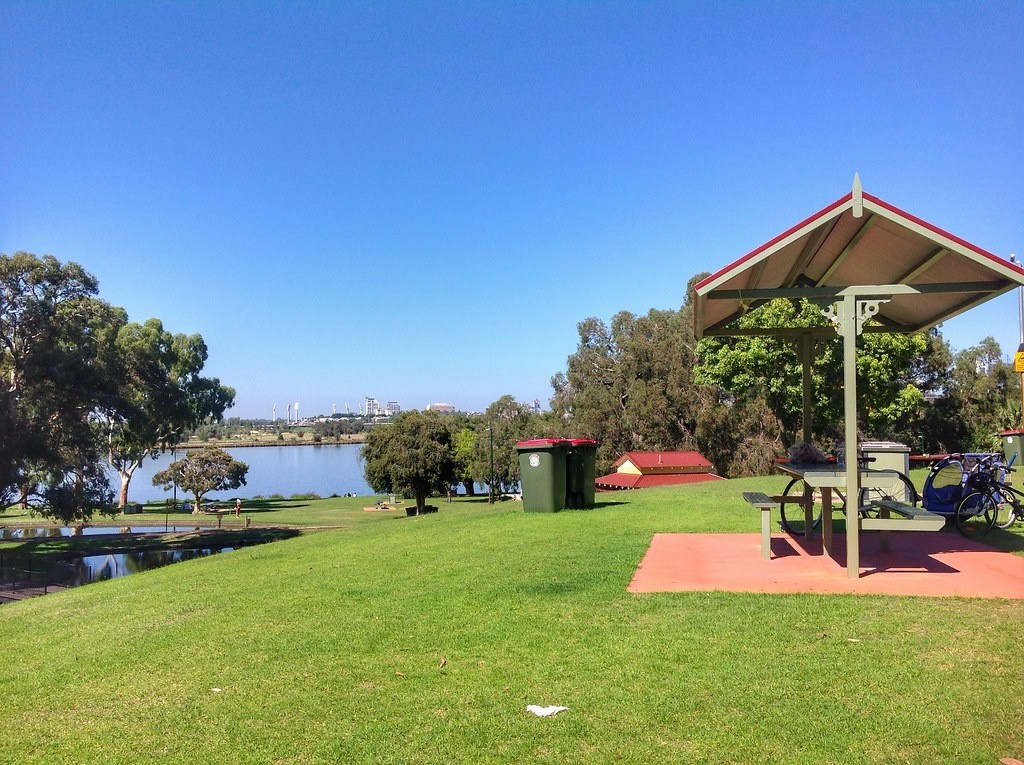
[694,171,1024,578]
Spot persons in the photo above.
[167,501,177,510]
[235,498,242,519]
[344,493,357,497]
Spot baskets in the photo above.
[966,470,991,491]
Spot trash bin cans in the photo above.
[562,438,599,510]
[121,504,136,514]
[513,439,572,513]
[134,504,142,514]
[425,505,439,513]
[999,429,1024,466]
[389,494,396,504]
[405,507,417,517]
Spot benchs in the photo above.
[742,491,805,559]
[857,499,946,550]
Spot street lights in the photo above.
[486,427,495,504]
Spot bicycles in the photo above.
[955,456,1024,538]
[780,449,918,537]
[979,456,1019,529]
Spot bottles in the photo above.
[837,448,846,471]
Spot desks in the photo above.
[776,461,898,555]
